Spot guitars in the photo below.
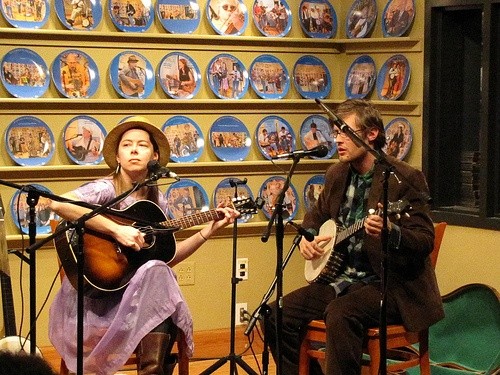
[68,145,99,161]
[166,74,194,93]
[306,139,333,149]
[120,76,144,95]
[176,202,201,216]
[1,194,45,359]
[303,198,415,285]
[53,194,259,300]
[388,140,407,155]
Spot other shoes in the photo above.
[67,19,74,23]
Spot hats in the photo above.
[127,55,139,64]
[102,116,171,168]
[82,124,93,136]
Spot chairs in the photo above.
[299,222,447,375]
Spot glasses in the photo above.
[331,125,365,140]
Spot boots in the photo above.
[137,332,169,375]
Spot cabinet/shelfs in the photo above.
[0,27,422,251]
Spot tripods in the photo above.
[199,176,259,375]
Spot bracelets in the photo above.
[199,231,208,241]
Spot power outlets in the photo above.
[236,258,249,280]
[175,262,194,286]
[236,303,247,325]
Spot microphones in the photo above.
[290,223,315,242]
[147,160,180,181]
[316,99,362,149]
[272,145,328,160]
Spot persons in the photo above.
[257,95,437,375]
[0,1,415,225]
[48,120,241,375]
